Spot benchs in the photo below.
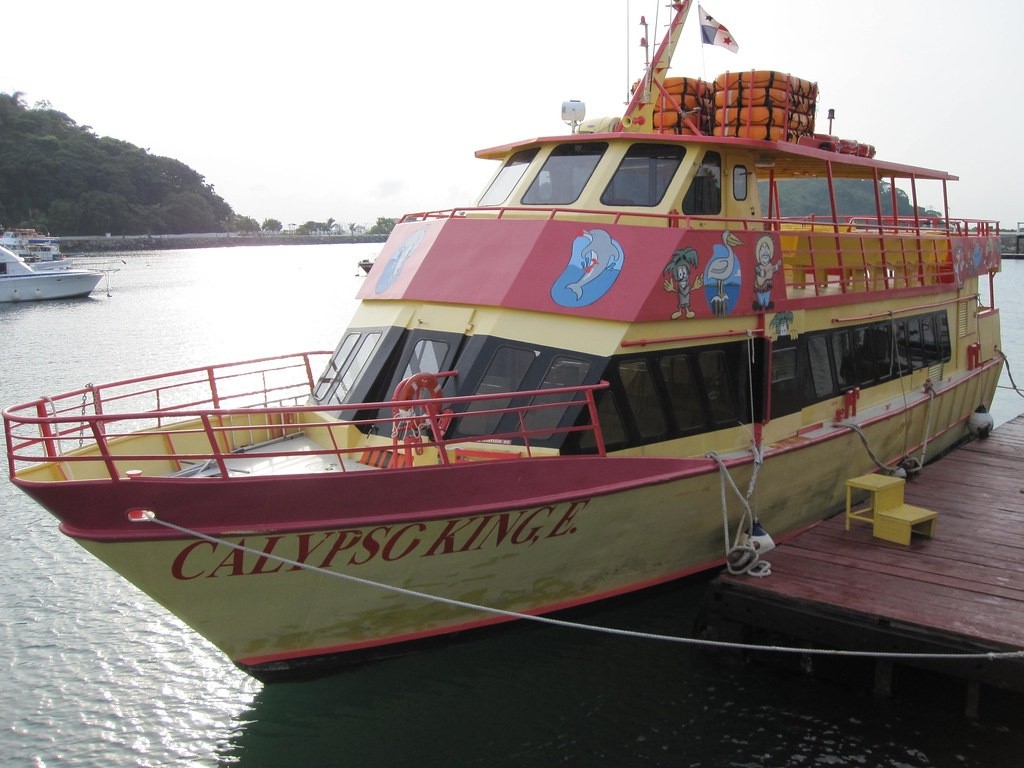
[778,223,948,291]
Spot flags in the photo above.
[698,5,739,52]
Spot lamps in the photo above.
[562,100,585,135]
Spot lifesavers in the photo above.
[399,372,442,429]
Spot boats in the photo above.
[358,259,373,273]
[0,1,1008,688]
[0,229,105,305]
